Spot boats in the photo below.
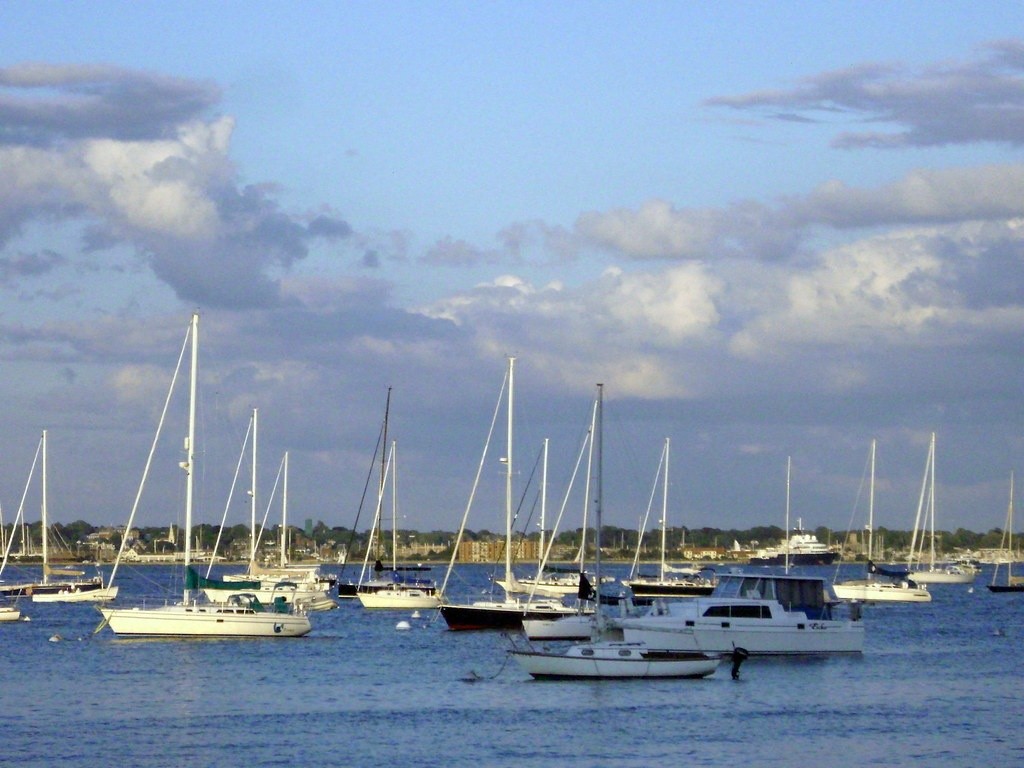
[748,517,838,566]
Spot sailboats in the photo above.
[0,310,1024,678]
